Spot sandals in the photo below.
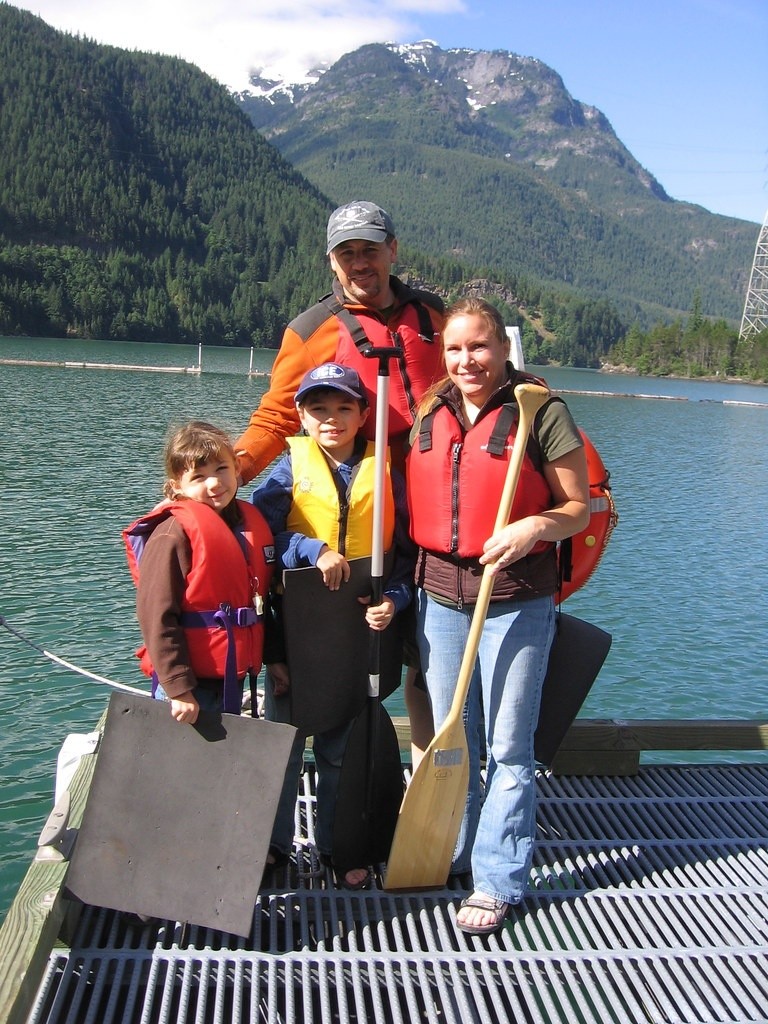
[319,851,371,892]
[455,891,508,935]
[263,846,289,875]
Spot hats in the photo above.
[326,200,395,255]
[293,362,364,402]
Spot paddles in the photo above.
[332,348,404,874]
[382,383,550,891]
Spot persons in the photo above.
[403,297,592,934]
[149,201,450,777]
[247,362,424,891]
[119,420,278,928]
